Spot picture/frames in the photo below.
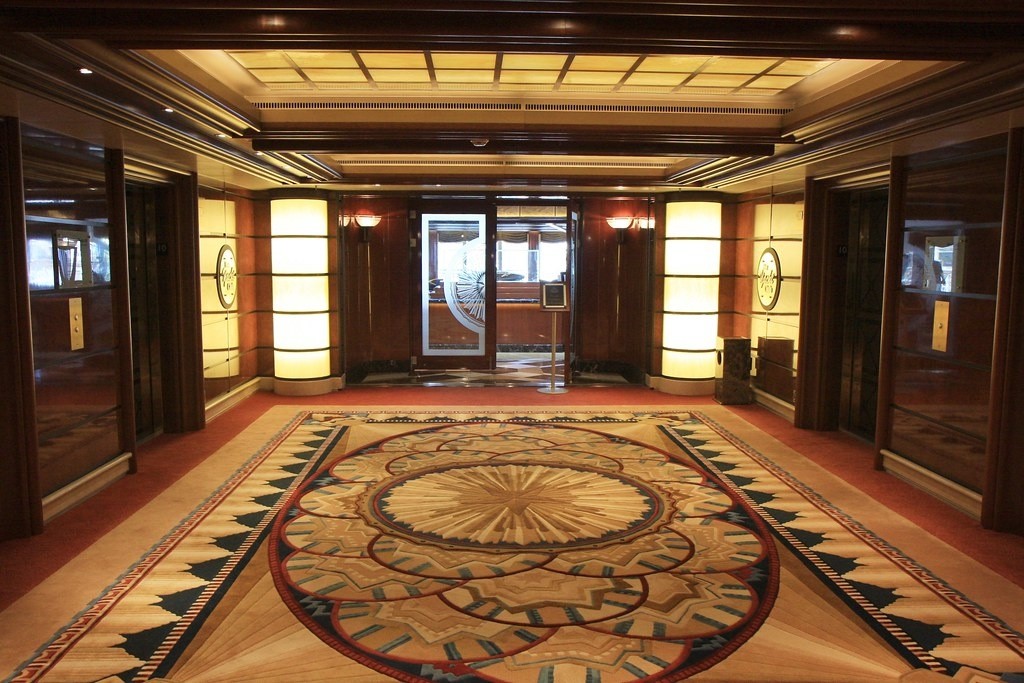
[539,280,571,312]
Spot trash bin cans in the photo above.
[712,334,752,406]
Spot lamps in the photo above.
[355,215,382,243]
[606,216,635,245]
